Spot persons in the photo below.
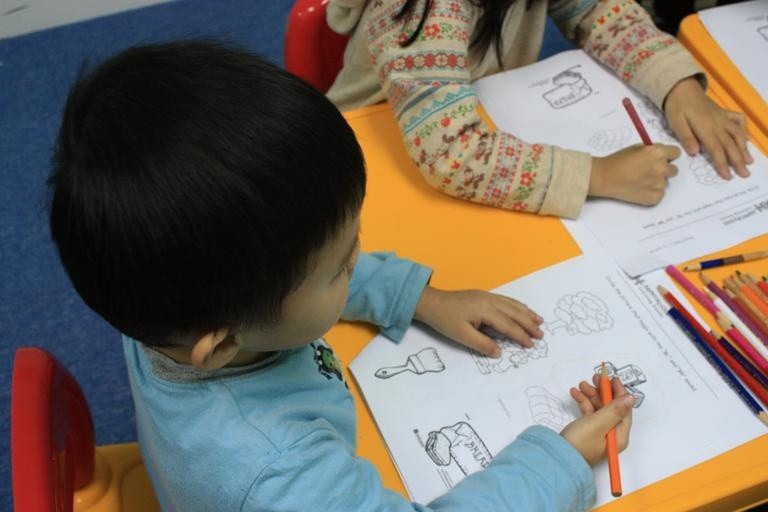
[319,0,755,221]
[46,39,634,511]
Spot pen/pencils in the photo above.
[623,99,651,146]
[599,363,620,496]
[657,252,768,425]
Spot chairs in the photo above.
[287,0,349,92]
[10,348,160,512]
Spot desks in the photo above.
[322,2,768,512]
